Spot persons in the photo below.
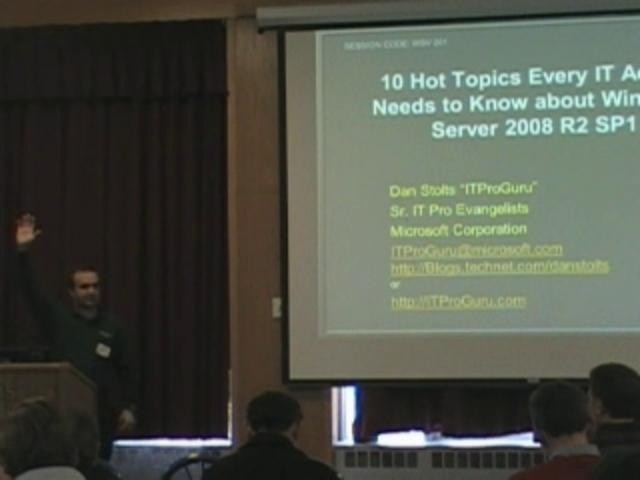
[15,211,137,462]
[202,389,341,479]
[60,405,123,480]
[513,381,605,480]
[586,361,639,452]
[0,398,86,480]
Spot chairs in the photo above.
[162,451,219,480]
